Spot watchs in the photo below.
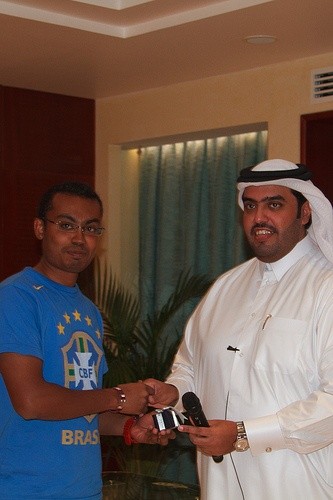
[233,421,249,452]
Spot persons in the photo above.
[144,159,333,500]
[0,183,175,500]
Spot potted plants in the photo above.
[74,256,216,500]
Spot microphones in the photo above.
[182,392,223,463]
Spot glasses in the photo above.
[38,213,104,238]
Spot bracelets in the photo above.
[124,416,138,445]
[113,387,126,411]
